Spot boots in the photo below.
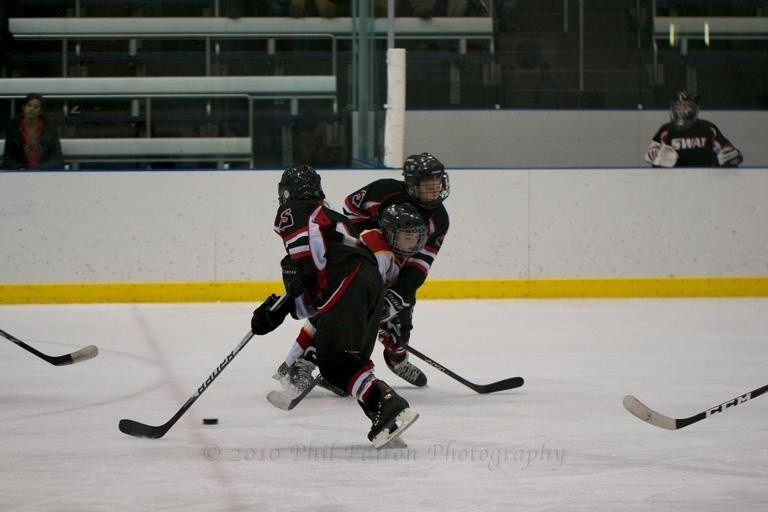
[288,346,317,391]
[384,350,426,386]
[359,379,409,440]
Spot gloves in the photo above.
[379,288,410,326]
[251,294,286,335]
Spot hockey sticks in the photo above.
[0,330,99,366]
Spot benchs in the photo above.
[644,14,766,71]
[0,13,495,169]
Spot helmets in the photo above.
[278,165,325,206]
[381,203,425,256]
[403,152,449,209]
[672,91,700,131]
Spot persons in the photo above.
[1,92,66,172]
[288,151,449,389]
[641,90,745,168]
[250,163,409,442]
